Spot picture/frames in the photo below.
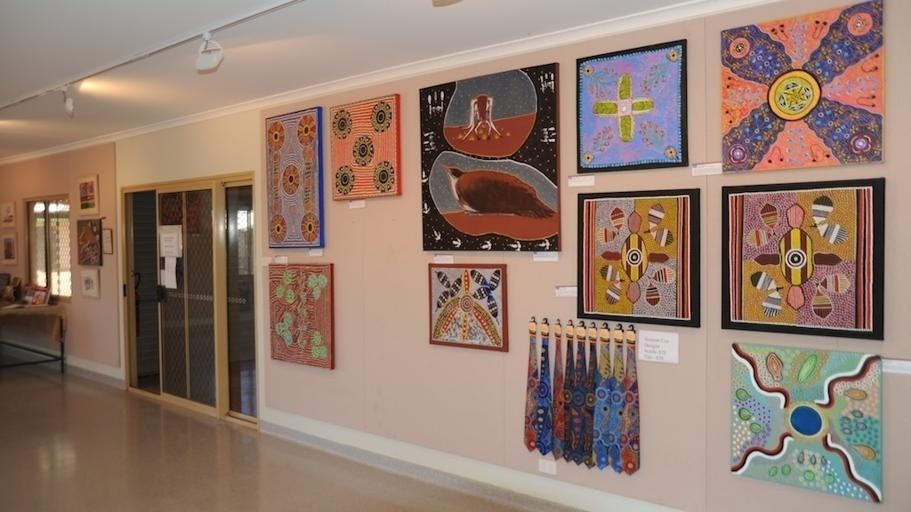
[577,39,688,174]
[721,176,886,341]
[428,263,510,352]
[1,176,114,307]
[578,188,700,329]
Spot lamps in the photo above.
[1,0,302,118]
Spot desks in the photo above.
[0,307,67,373]
